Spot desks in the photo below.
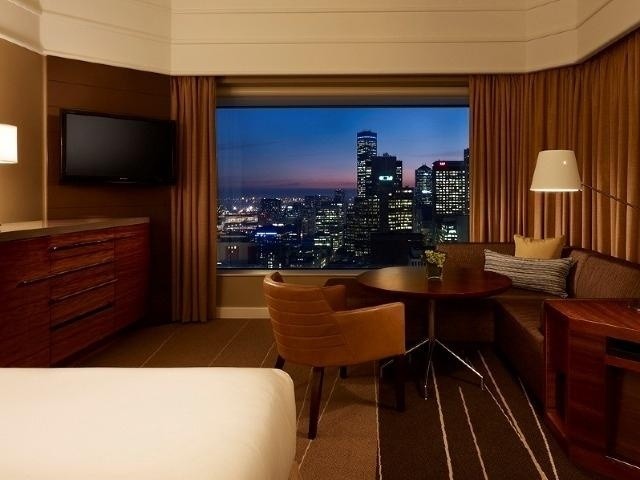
[356,266,513,401]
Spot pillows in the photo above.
[482,233,578,299]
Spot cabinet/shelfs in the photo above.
[541,298,640,480]
[0,217,151,367]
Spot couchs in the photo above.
[435,243,640,407]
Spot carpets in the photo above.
[376,338,596,479]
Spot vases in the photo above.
[426,262,443,280]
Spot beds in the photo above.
[0,367,298,480]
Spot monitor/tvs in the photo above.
[56,108,182,189]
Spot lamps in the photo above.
[530,149,639,213]
[0,124,19,165]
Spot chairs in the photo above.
[263,269,409,439]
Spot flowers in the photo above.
[424,249,447,277]
